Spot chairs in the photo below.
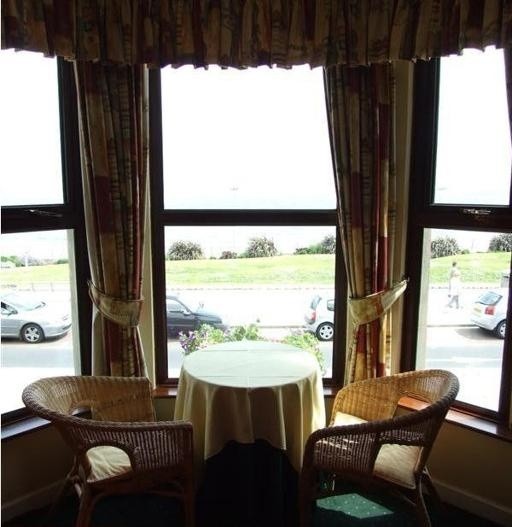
[294,368,460,527]
[21,374,197,526]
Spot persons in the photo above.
[446,261,461,310]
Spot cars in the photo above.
[165,294,225,338]
[0,291,72,345]
[467,269,510,339]
[304,291,335,342]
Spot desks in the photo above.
[180,340,320,457]
